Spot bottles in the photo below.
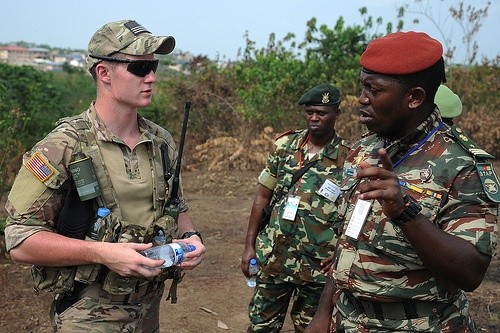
[139,242,196,269]
[246,259,259,287]
[88,208,111,241]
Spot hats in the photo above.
[358,32,443,76]
[299,84,342,105]
[435,85,462,118]
[87,20,176,70]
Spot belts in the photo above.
[112,281,157,304]
[359,301,437,321]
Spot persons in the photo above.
[5,20,205,333]
[433,85,462,126]
[241,84,351,333]
[305,31,500,333]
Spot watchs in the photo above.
[390,194,422,227]
[180,229,204,245]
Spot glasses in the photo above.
[89,55,159,77]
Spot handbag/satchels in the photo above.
[258,206,274,228]
